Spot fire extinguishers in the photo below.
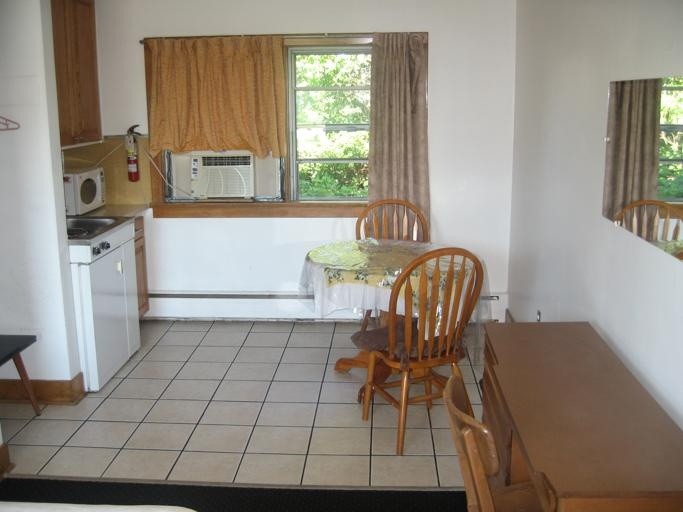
[127,124,142,182]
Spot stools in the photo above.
[0,334,42,416]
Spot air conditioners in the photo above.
[190,150,256,201]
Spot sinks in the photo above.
[65,213,135,245]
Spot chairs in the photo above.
[362,245,484,454]
[355,199,429,336]
[443,362,539,512]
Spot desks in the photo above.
[307,240,476,405]
[481,321,683,512]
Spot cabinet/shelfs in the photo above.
[50,0,103,147]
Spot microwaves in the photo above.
[62,166,107,218]
